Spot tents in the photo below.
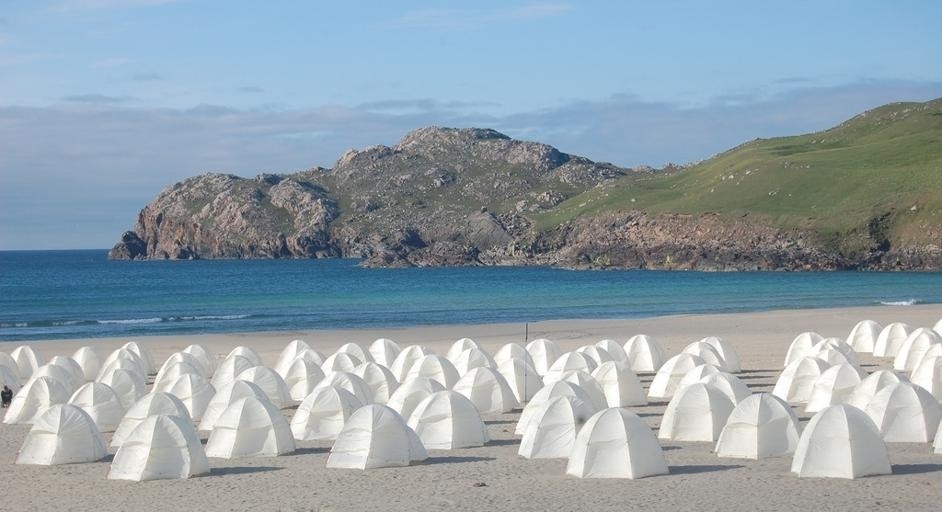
[73,341,156,412]
[770,319,941,479]
[153,344,293,411]
[275,339,490,472]
[106,372,216,482]
[197,377,296,461]
[453,339,669,479]
[624,334,805,462]
[1,343,48,398]
[1,355,127,466]
